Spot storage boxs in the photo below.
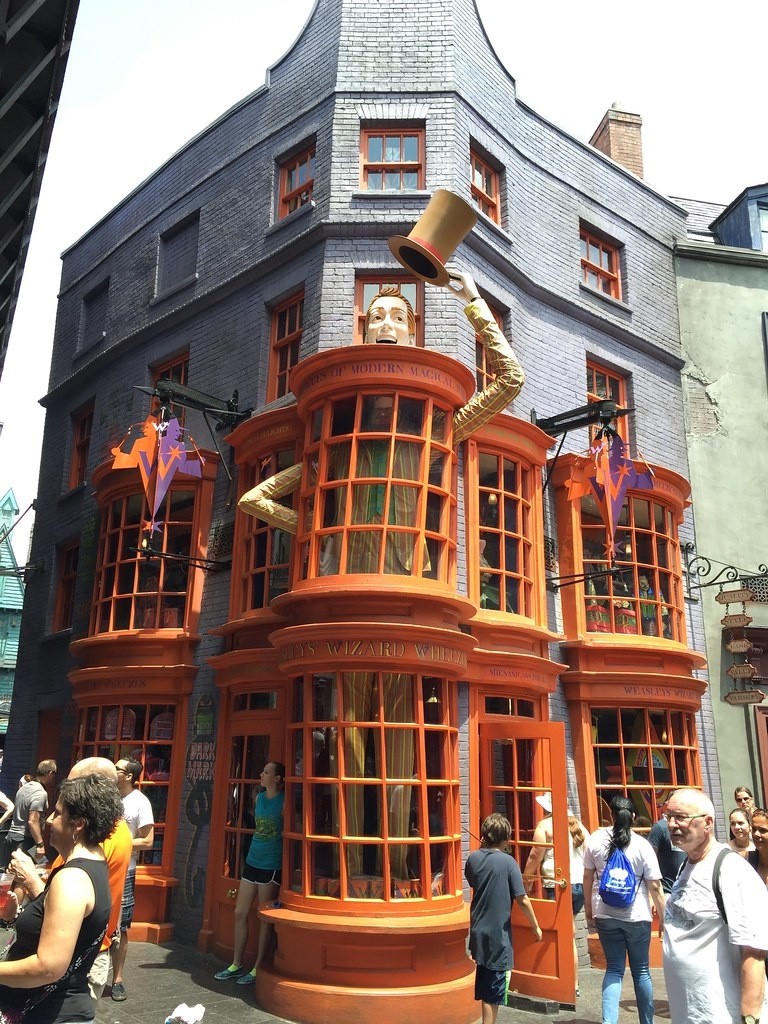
[142,607,183,628]
[314,872,446,898]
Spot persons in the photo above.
[238,268,524,876]
[214,761,285,984]
[583,798,666,1024]
[663,789,768,1024]
[0,755,154,1024]
[726,787,768,980]
[648,819,687,905]
[524,792,590,999]
[464,812,543,1024]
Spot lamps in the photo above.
[485,491,498,519]
[426,678,442,705]
[622,503,632,557]
[129,531,232,573]
[661,707,668,744]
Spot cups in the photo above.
[0,874,16,908]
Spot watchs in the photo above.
[36,842,44,847]
[740,1015,760,1024]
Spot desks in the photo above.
[480,582,514,613]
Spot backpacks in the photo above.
[596,829,644,907]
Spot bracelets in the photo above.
[3,904,22,926]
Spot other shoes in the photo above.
[575,989,579,999]
[214,966,246,979]
[237,974,257,983]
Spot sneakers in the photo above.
[110,981,127,1000]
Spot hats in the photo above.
[535,791,575,816]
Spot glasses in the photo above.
[736,797,751,802]
[116,767,128,772]
[48,771,57,775]
[663,814,708,822]
